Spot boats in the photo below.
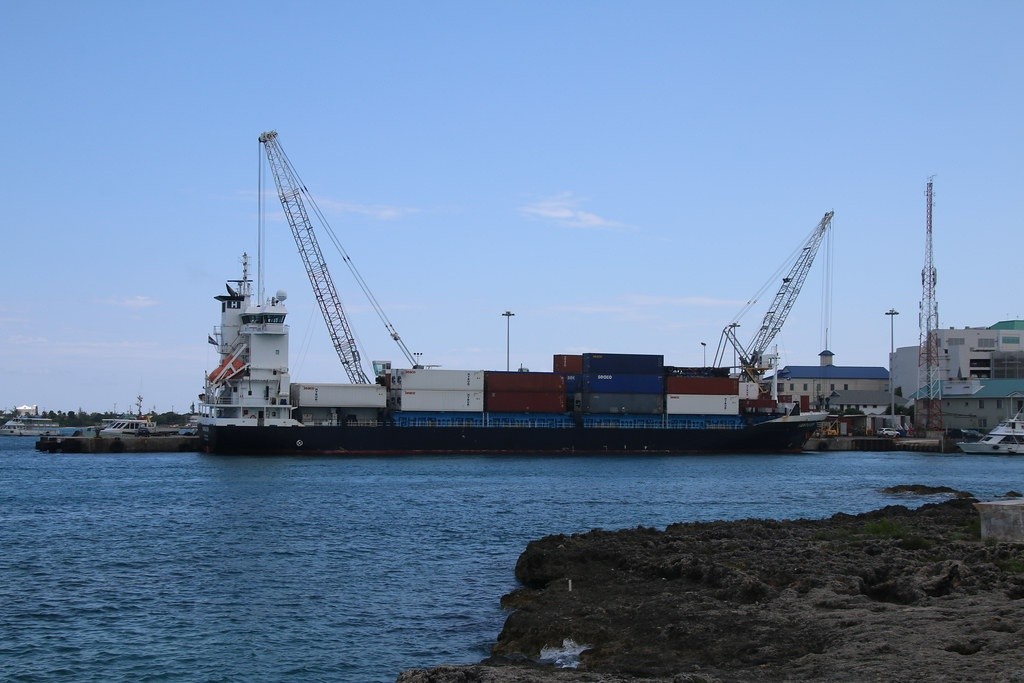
[956,407,1024,453]
[0,418,60,436]
[190,253,830,457]
[98,420,156,434]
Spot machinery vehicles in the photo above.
[713,211,835,383]
[258,130,425,388]
[823,418,839,438]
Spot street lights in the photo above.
[885,308,899,428]
[728,323,740,377]
[826,328,828,350]
[502,311,515,371]
[701,342,706,367]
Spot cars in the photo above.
[134,428,150,438]
[944,429,978,439]
[877,428,900,438]
